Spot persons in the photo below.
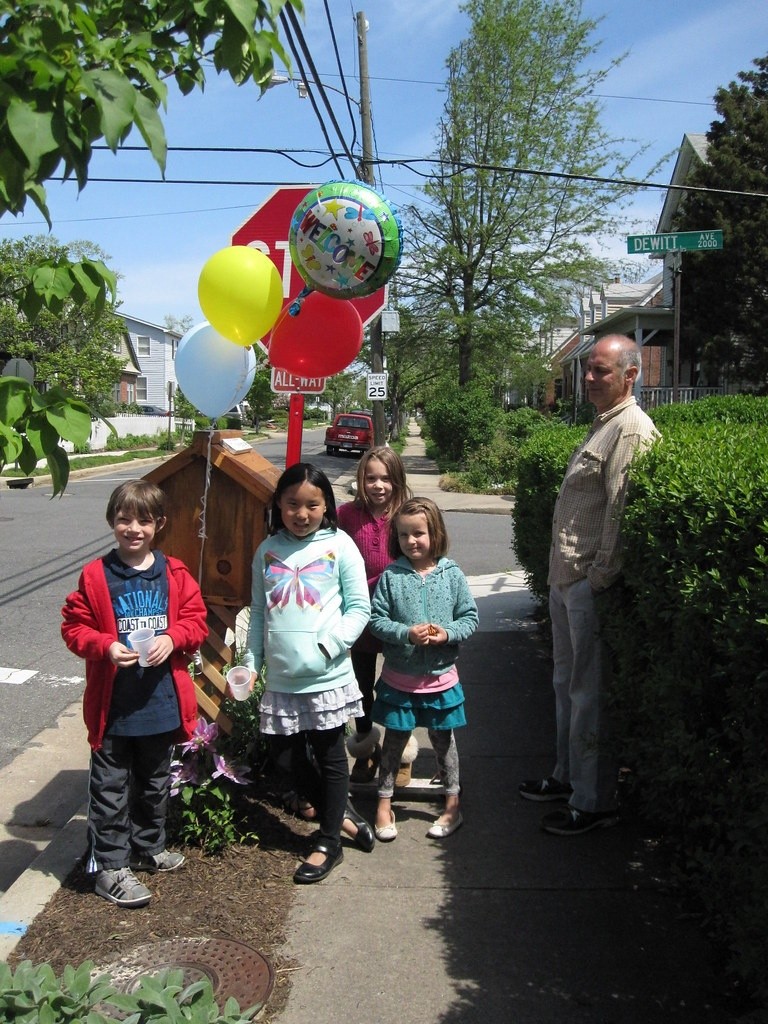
[518,334,663,835]
[61,480,209,907]
[239,445,479,884]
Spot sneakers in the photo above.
[518,776,574,802]
[95,866,151,908]
[538,804,621,835]
[130,849,185,872]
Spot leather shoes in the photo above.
[293,844,344,884]
[341,799,375,852]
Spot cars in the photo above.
[195,404,243,425]
[137,404,168,417]
[323,412,374,458]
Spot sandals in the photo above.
[279,792,317,822]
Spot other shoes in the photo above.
[428,810,463,838]
[374,810,398,841]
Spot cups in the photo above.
[127,628,155,667]
[227,666,251,702]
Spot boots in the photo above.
[394,735,419,786]
[347,726,381,783]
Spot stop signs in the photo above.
[227,188,388,356]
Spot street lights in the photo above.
[315,394,319,425]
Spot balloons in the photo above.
[173,182,402,421]
